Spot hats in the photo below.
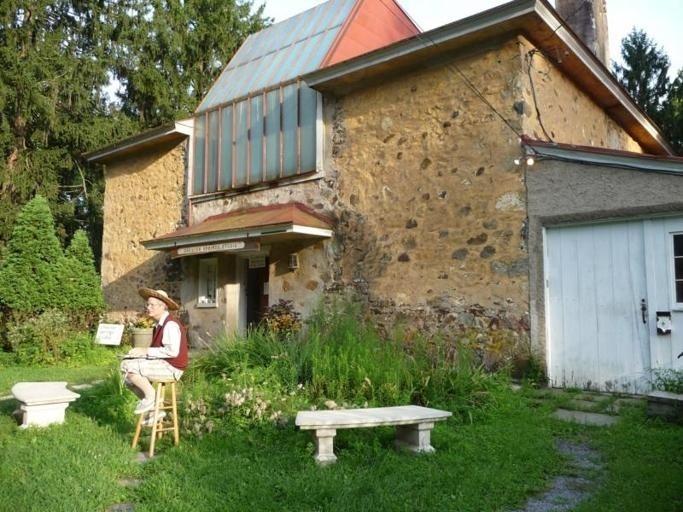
[139,288,180,310]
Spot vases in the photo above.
[131,328,153,346]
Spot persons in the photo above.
[119,296,188,425]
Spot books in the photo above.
[121,353,148,359]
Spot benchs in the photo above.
[293,403,451,463]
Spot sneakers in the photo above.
[142,408,166,424]
[134,399,154,415]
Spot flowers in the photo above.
[130,318,156,329]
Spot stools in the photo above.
[130,379,180,458]
